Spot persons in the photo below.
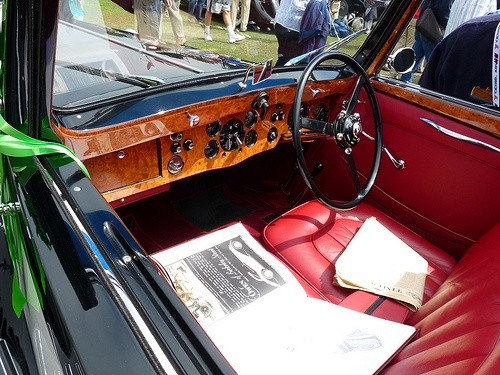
[231,0,251,31]
[418,14,500,113]
[365,0,377,34]
[188,0,205,24]
[204,0,245,44]
[134,0,172,41]
[161,0,186,45]
[274,0,329,68]
[443,0,498,41]
[383,0,454,83]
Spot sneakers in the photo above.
[230,34,245,43]
[175,37,186,47]
[204,31,212,41]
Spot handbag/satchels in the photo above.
[415,8,444,43]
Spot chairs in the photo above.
[262,190,500,339]
[153,221,500,375]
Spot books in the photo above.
[147,220,420,375]
